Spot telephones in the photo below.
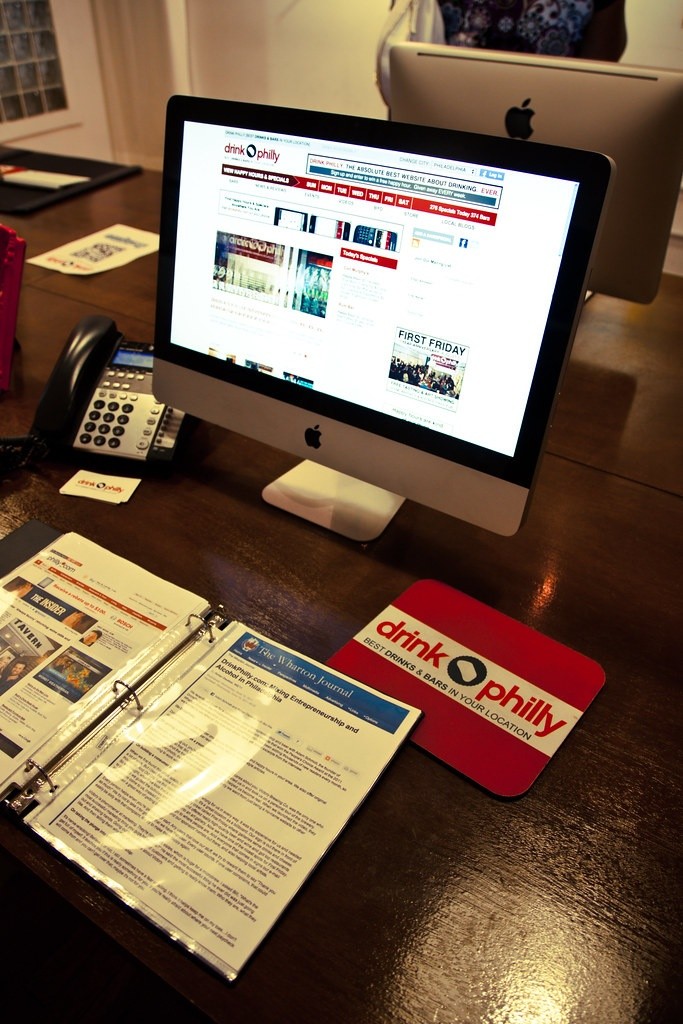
[24,314,190,479]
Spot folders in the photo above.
[0,531,422,986]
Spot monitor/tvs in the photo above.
[387,43,682,303]
[151,94,614,536]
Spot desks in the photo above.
[0,145,681,1024]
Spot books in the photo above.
[0,519,425,982]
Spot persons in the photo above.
[78,629,102,647]
[0,654,11,670]
[0,661,27,696]
[55,660,72,674]
[387,354,459,398]
